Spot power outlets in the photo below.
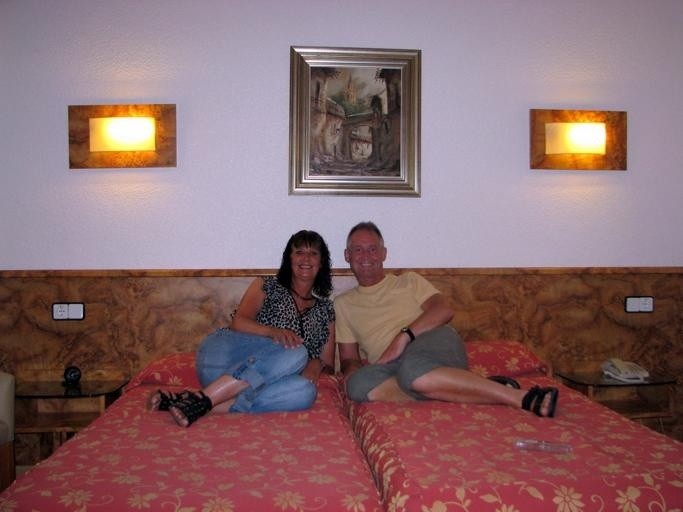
[52,304,66,318]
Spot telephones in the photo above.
[600,358,650,379]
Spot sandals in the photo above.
[167,389,214,429]
[485,375,521,389]
[521,385,560,419]
[145,387,194,414]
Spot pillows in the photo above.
[119,353,342,401]
[463,338,548,378]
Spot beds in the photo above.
[335,339,682,511]
[3,339,386,511]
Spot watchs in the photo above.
[401,327,415,341]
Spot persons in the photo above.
[150,229,335,427]
[332,221,559,417]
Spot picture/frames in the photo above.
[289,47,422,197]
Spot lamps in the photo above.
[66,103,177,167]
[529,108,627,170]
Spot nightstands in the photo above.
[557,371,675,422]
[15,378,125,434]
